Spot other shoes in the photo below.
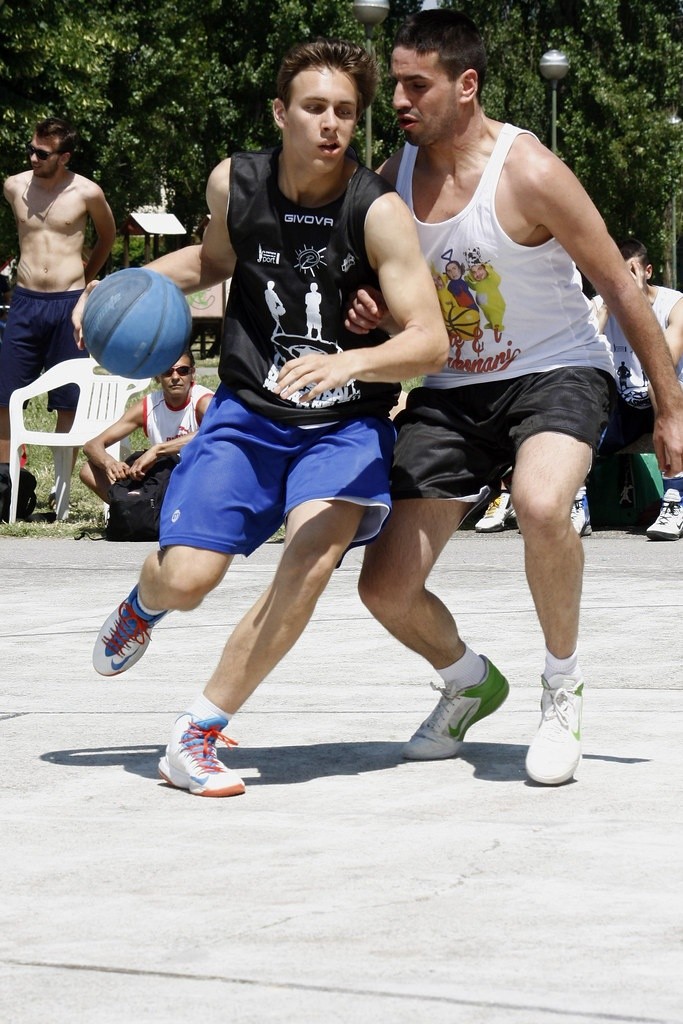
[49,492,72,517]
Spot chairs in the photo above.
[8,358,153,526]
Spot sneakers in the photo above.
[570,486,592,538]
[525,674,584,784]
[400,654,510,761]
[157,715,245,796]
[474,492,517,533]
[92,583,176,677]
[646,488,683,541]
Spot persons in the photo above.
[70,35,451,797]
[0,116,117,515]
[78,348,216,505]
[587,238,683,541]
[343,8,683,787]
[472,477,592,537]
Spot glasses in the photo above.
[162,362,195,376]
[25,139,63,160]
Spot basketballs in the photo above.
[81,267,194,379]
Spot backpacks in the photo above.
[106,449,181,542]
[0,462,37,524]
